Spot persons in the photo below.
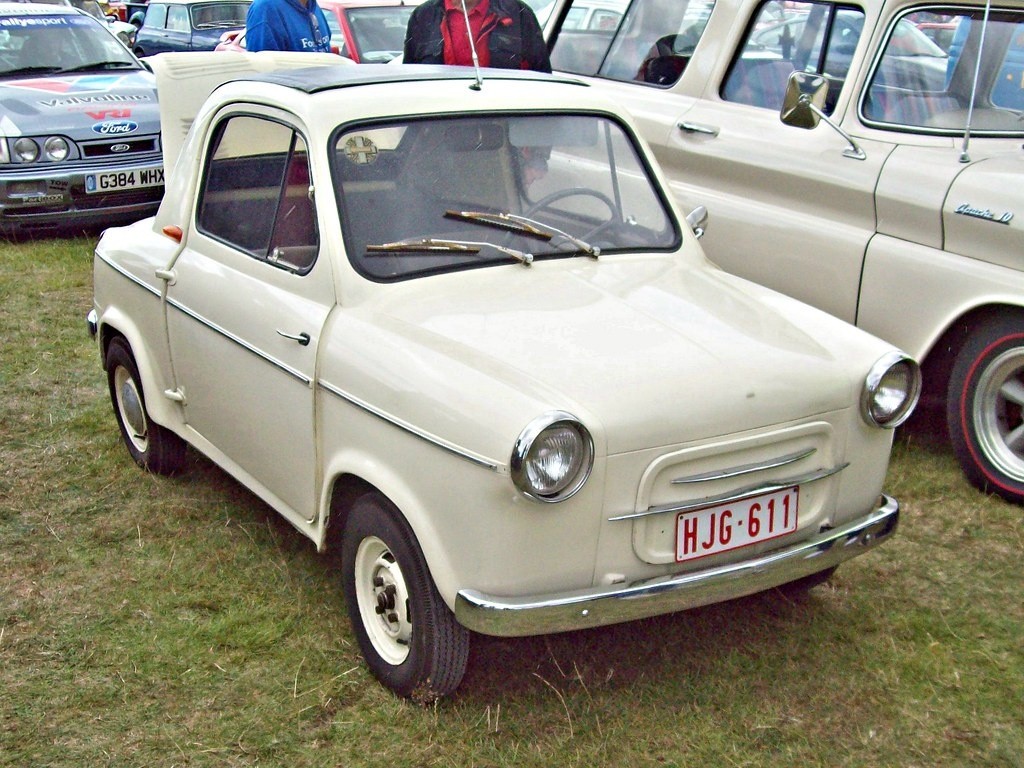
[404,0,553,188]
[244,0,332,53]
[30,29,79,69]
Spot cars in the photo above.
[525,0,1024,130]
[87,65,923,707]
[508,0,1024,504]
[1,0,256,59]
[214,0,428,66]
[1,2,165,241]
